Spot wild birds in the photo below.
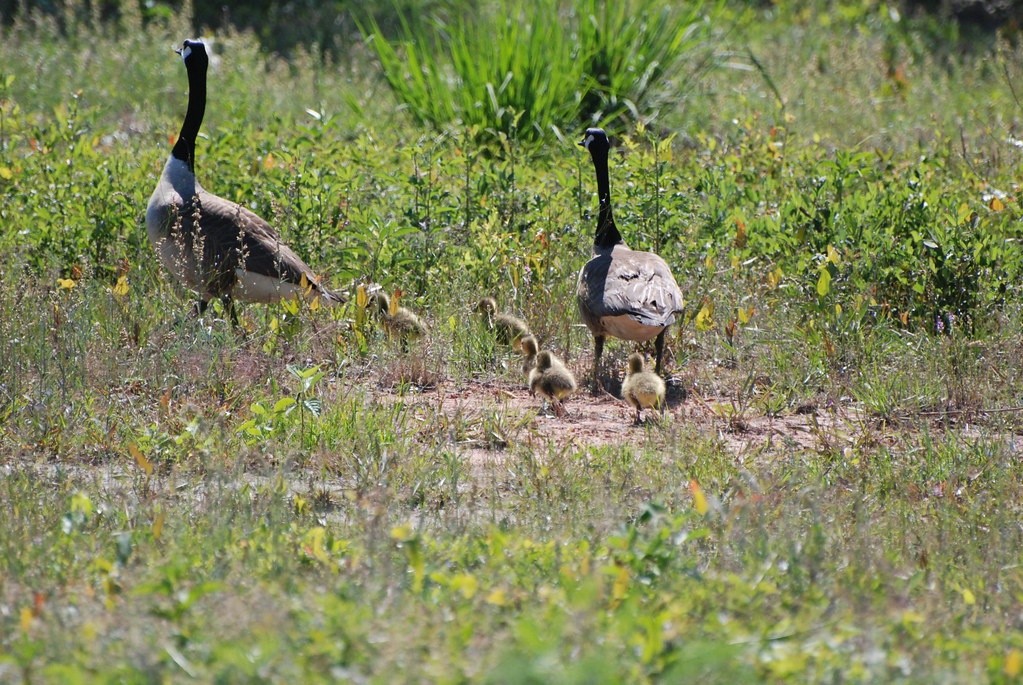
[620,353,671,426]
[143,39,347,343]
[575,127,687,394]
[371,292,577,409]
